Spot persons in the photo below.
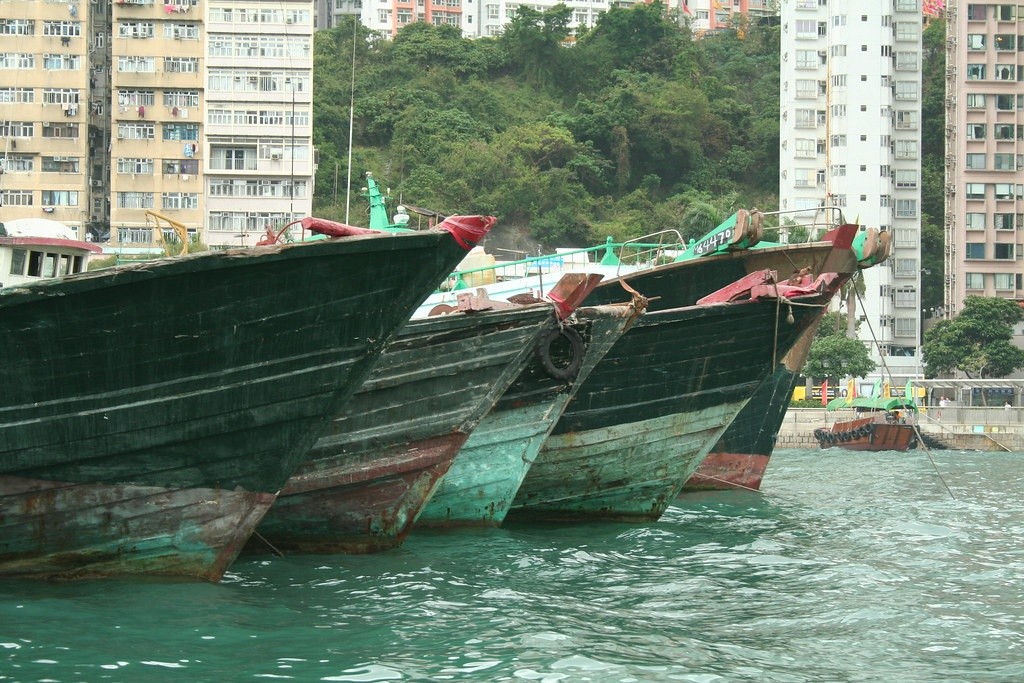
[939,396,951,407]
[1005,400,1013,410]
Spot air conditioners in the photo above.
[90,215,100,222]
[93,181,102,187]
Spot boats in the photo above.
[498,272,851,524]
[0,216,499,586]
[814,396,921,453]
[414,301,646,532]
[236,274,604,563]
[0,216,103,288]
[518,220,894,491]
